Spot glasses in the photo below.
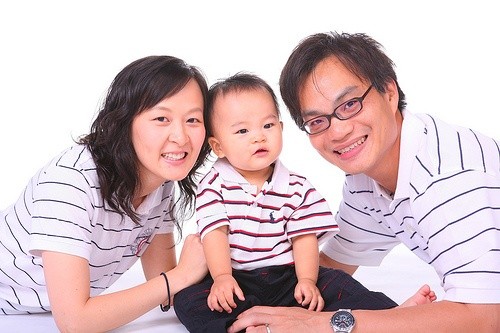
[298,84,374,135]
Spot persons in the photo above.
[173,71,437,333]
[0,54,209,333]
[227,32,500,333]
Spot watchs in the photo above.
[330,309,355,333]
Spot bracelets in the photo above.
[160,272,171,312]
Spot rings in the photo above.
[267,324,270,333]
